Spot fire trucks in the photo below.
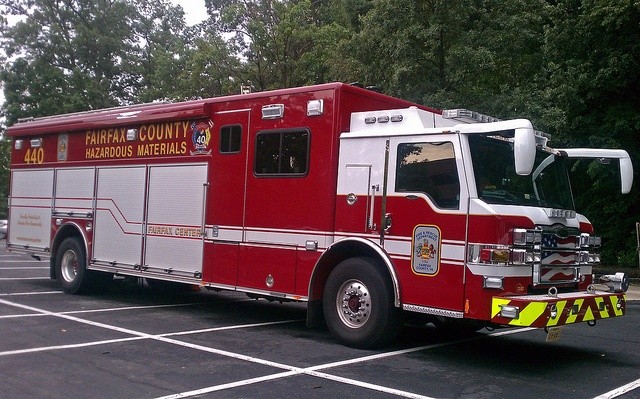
[5,82,634,350]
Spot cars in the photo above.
[0,219,8,234]
